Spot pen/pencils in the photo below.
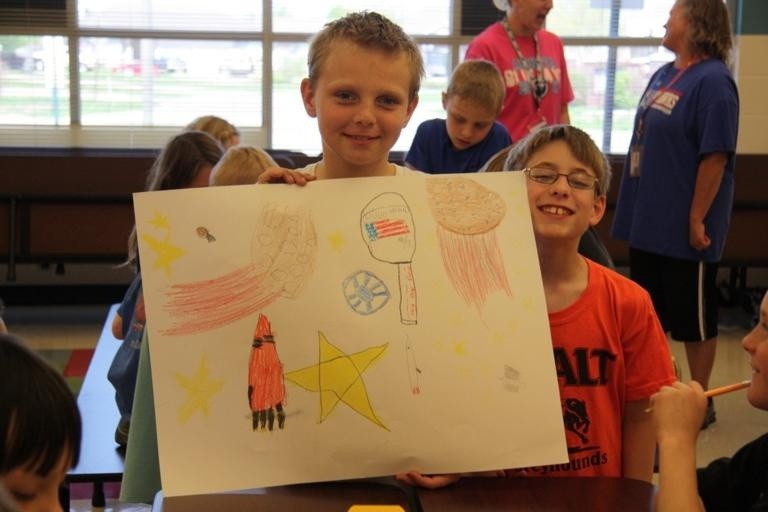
[643,380,751,414]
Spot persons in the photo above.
[107,2,768,512]
[1,333,83,512]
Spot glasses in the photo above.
[522,167,600,189]
